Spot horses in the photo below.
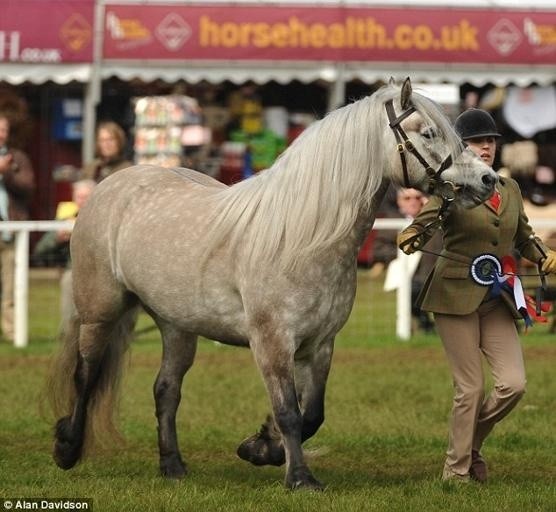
[38,75,500,493]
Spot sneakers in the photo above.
[468,448,490,484]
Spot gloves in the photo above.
[540,247,556,274]
[396,226,423,257]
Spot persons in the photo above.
[399,107,556,490]
[1,83,554,345]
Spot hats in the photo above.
[450,106,503,143]
